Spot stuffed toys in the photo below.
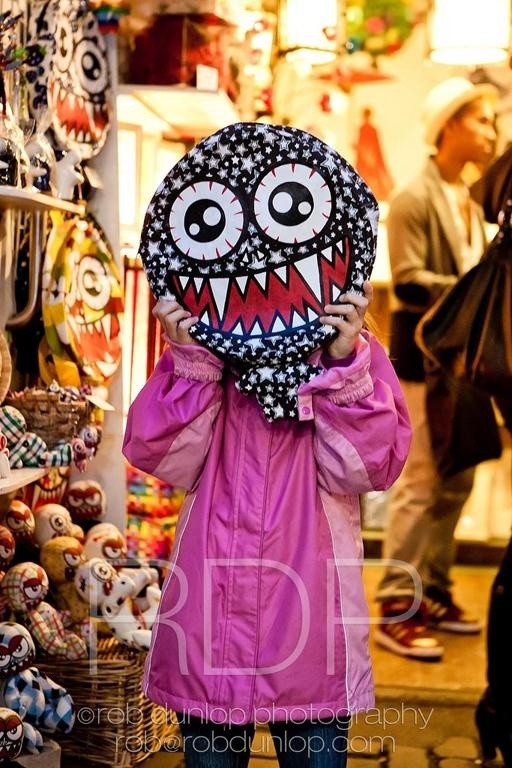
[0,383,163,768]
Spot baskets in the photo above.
[31,652,178,768]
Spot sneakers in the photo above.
[417,591,483,634]
[376,599,445,660]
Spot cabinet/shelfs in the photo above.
[0,183,88,766]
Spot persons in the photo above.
[0,383,163,768]
[373,76,498,661]
[120,298,411,768]
[470,131,512,767]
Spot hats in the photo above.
[422,76,498,144]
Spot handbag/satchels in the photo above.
[414,226,506,394]
